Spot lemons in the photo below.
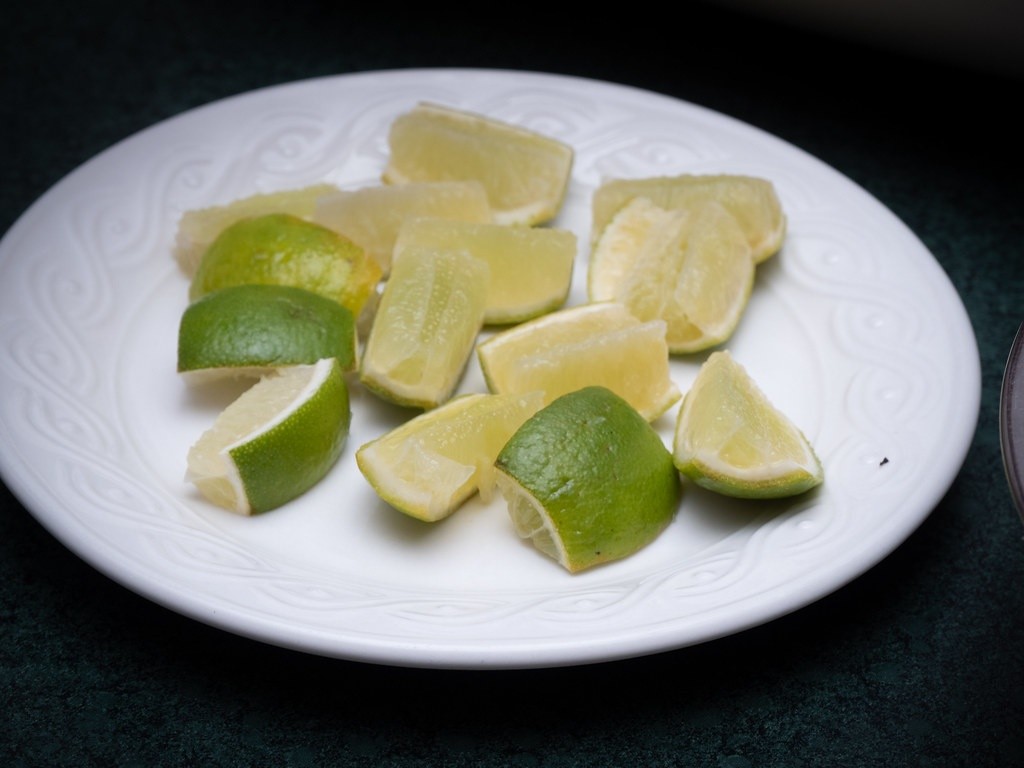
[174,102,825,572]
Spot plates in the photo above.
[999,318,1024,527]
[0,66,981,670]
